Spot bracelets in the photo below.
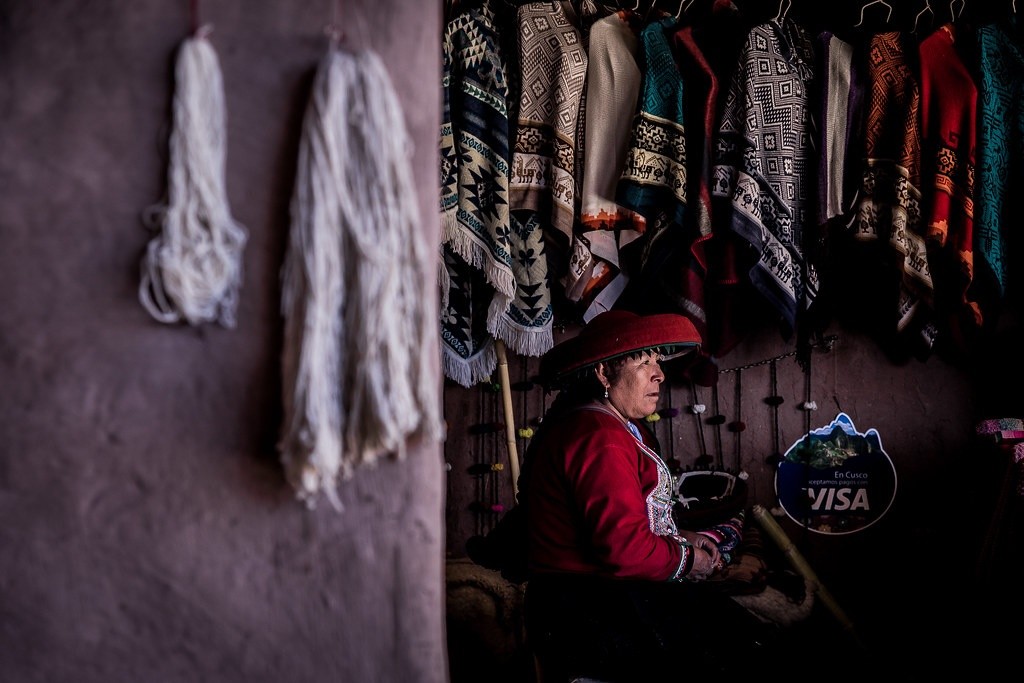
[680,545,696,581]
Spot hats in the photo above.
[538,310,703,399]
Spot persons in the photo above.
[516,312,744,683]
